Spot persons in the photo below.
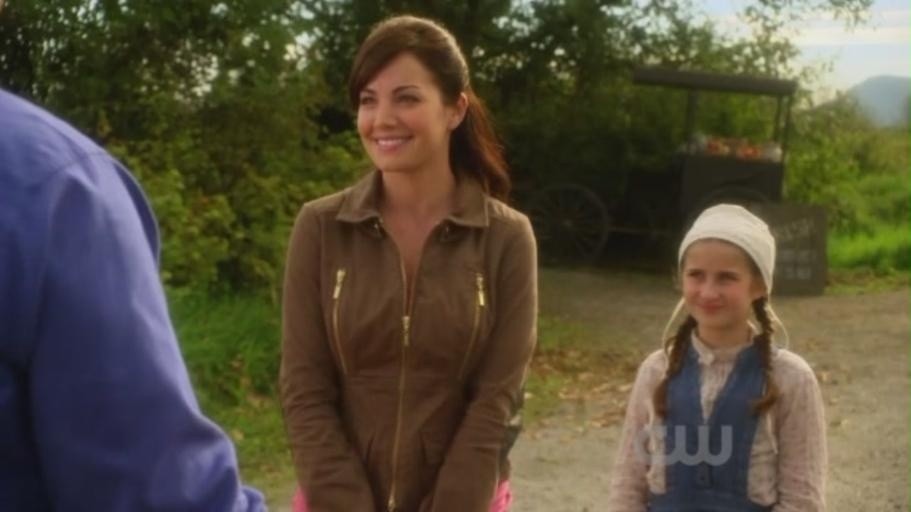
[274,17,539,512]
[607,203,825,511]
[1,89,266,511]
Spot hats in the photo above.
[678,204,775,296]
